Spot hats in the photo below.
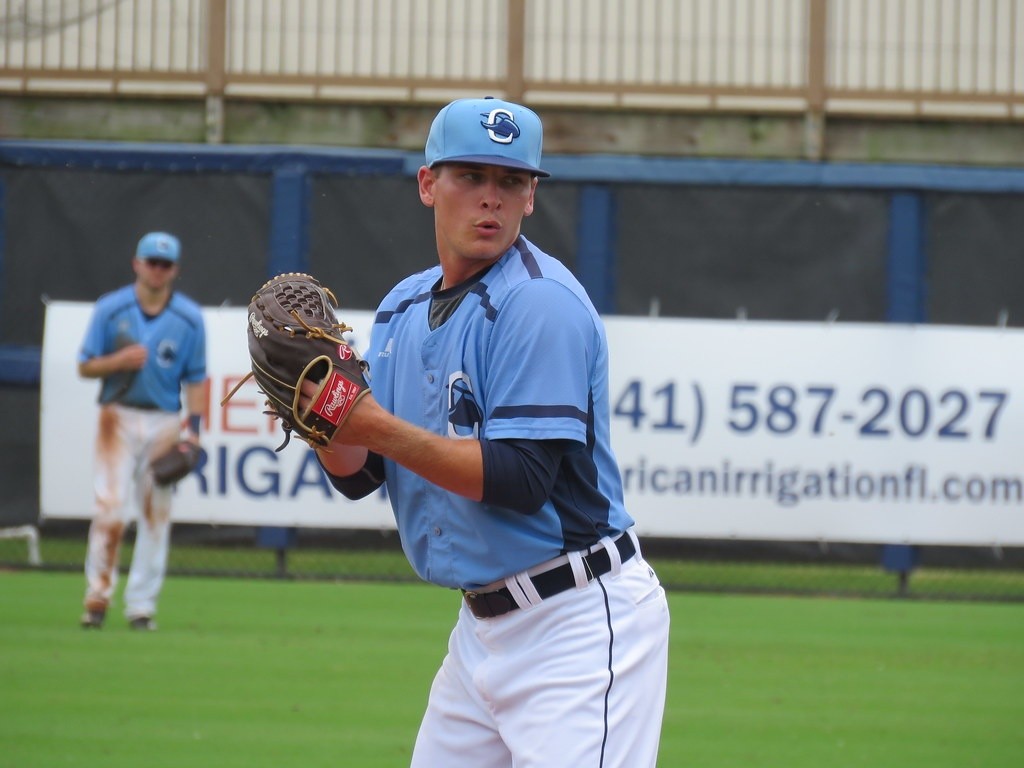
[424,96,552,178]
[137,232,181,262]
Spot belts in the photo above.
[460,532,636,620]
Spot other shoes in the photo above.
[130,618,156,631]
[80,607,104,629]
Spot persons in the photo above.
[77,231,207,629]
[249,98,670,768]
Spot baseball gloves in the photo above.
[149,437,209,487]
[242,270,371,449]
